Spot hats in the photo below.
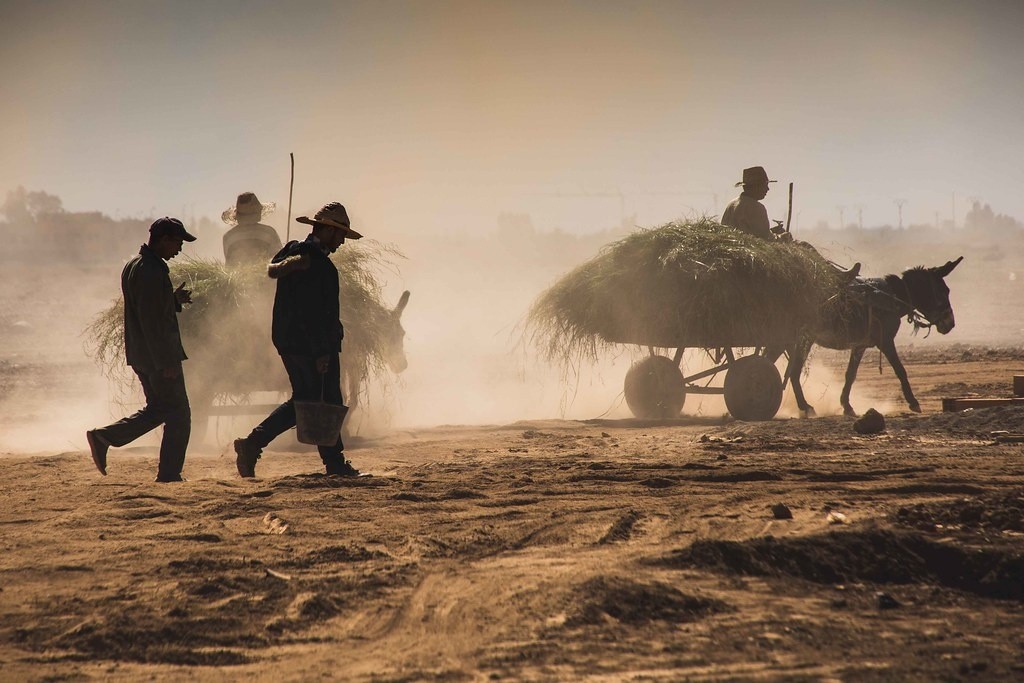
[734,166,778,188]
[220,191,278,226]
[149,217,197,242]
[295,202,363,240]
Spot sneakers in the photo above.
[234,437,262,477]
[326,461,372,477]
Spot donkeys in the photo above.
[762,256,965,420]
[342,289,408,444]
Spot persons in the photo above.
[233,199,372,477]
[85,216,199,483]
[222,192,282,269]
[721,166,861,284]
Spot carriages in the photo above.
[169,260,410,446]
[609,255,963,423]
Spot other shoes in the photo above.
[841,263,862,278]
[87,428,109,476]
[154,473,185,482]
